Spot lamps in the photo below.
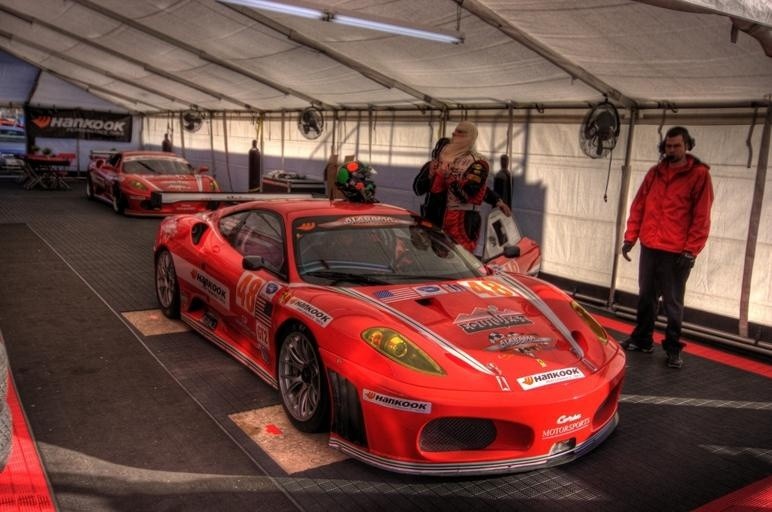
[226,0,465,45]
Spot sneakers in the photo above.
[668,351,682,369]
[620,336,656,353]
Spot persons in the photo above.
[412,136,513,232]
[614,125,714,369]
[431,121,490,255]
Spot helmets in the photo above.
[334,161,380,203]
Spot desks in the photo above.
[14,154,71,191]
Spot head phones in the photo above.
[659,138,694,153]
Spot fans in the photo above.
[180,105,203,132]
[298,103,323,139]
[580,99,620,159]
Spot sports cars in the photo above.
[85,147,227,220]
[0,116,25,136]
[0,151,24,172]
[145,182,627,477]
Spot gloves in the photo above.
[622,245,632,261]
[676,255,691,273]
